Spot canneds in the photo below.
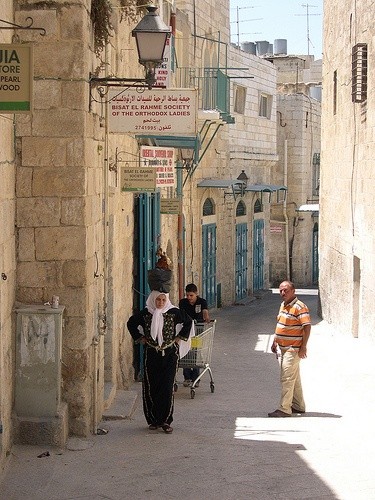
[52,295,59,309]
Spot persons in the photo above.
[178,283,210,388]
[126,289,193,433]
[268,280,311,418]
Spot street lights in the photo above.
[223,168,249,205]
[145,53,163,57]
[85,3,174,112]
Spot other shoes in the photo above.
[149,425,162,430]
[163,426,173,432]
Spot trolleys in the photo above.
[174,319,217,399]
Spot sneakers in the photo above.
[183,380,200,387]
[268,410,291,417]
[290,407,305,414]
